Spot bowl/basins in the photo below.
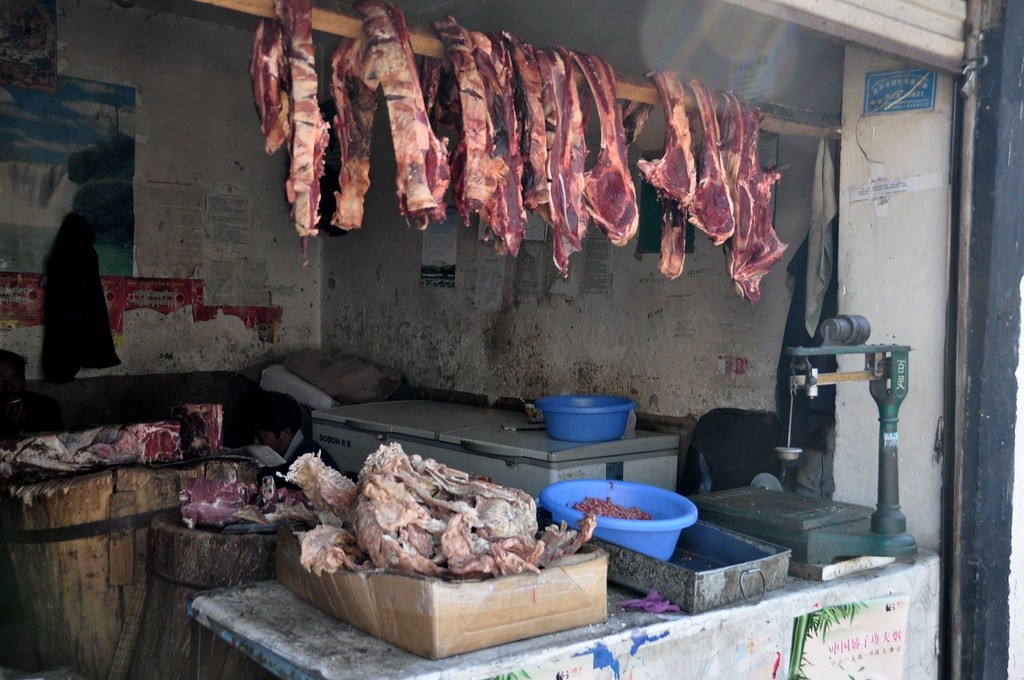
[535,476,699,561]
[532,396,638,439]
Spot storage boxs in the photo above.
[275,515,609,661]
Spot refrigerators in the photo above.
[310,393,684,507]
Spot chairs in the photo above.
[681,407,795,497]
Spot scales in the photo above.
[680,312,916,581]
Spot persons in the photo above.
[249,394,341,507]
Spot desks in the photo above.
[188,547,940,679]
